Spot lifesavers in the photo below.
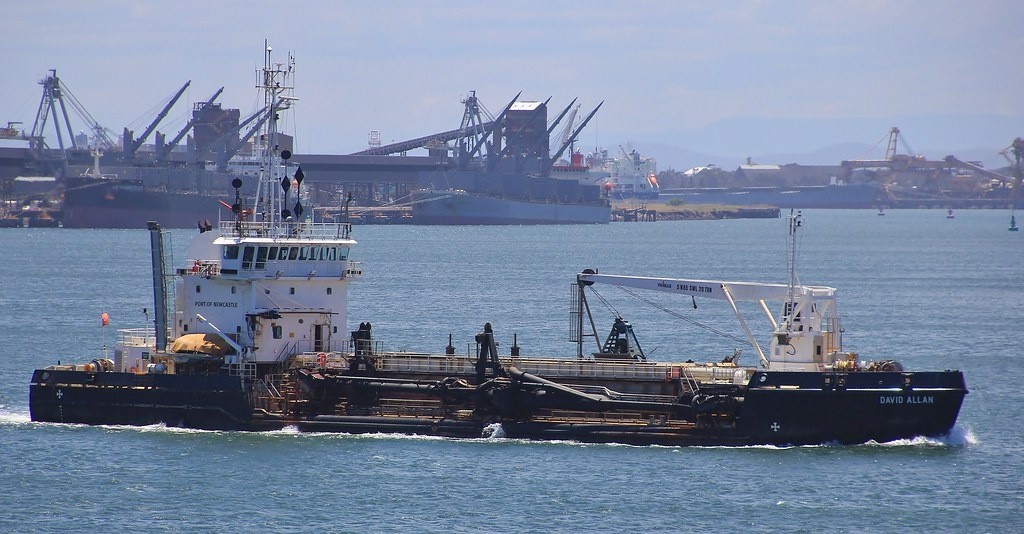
[317,353,327,366]
[442,377,460,394]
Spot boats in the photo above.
[32,40,971,445]
[36,70,288,229]
[411,97,613,224]
